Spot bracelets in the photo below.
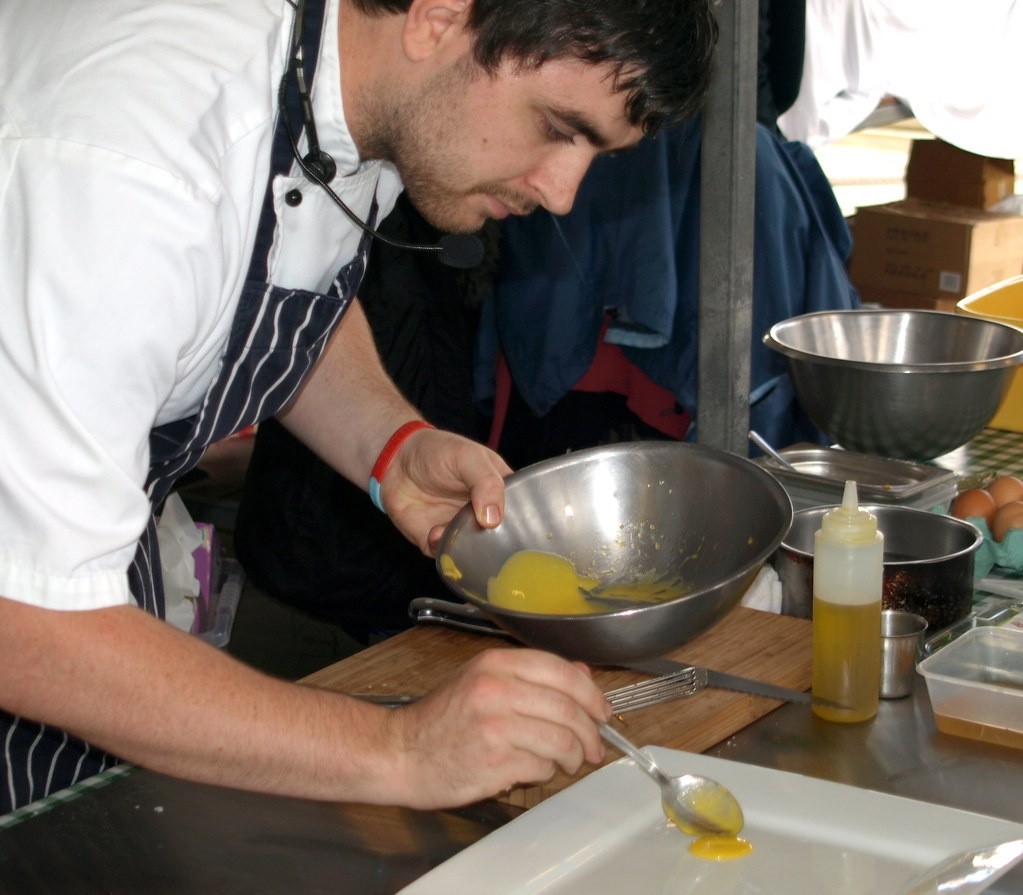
[370,421,435,513]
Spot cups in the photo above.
[878,608,930,700]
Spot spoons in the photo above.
[592,717,745,841]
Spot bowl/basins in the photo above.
[759,307,1023,460]
[915,625,1023,752]
[748,441,959,515]
[435,442,794,663]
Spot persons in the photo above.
[0,0,722,817]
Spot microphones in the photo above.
[319,180,484,268]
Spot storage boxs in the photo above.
[847,195,1023,315]
[905,139,1015,212]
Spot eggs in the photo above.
[949,476,1023,544]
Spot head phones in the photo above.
[295,0,336,186]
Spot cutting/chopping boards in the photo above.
[295,603,813,808]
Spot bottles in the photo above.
[808,477,879,725]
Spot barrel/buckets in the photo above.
[772,500,984,624]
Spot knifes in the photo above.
[407,595,858,714]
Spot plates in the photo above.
[391,743,1022,895]
